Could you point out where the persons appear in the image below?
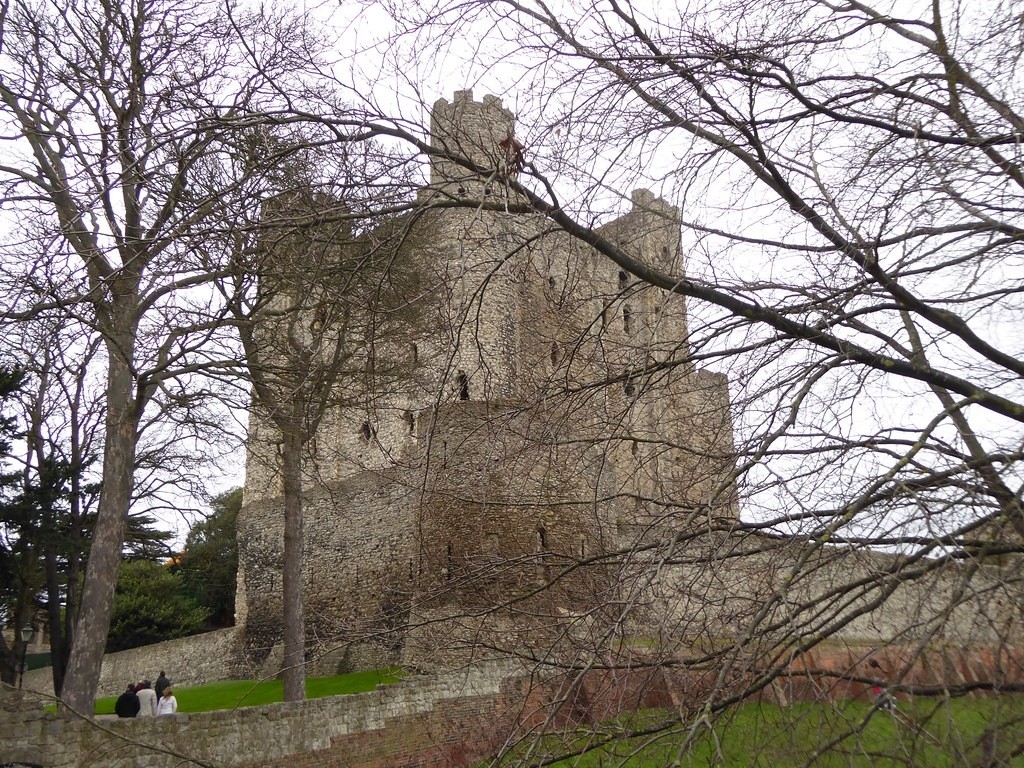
[115,671,177,718]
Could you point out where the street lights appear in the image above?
[18,622,35,691]
